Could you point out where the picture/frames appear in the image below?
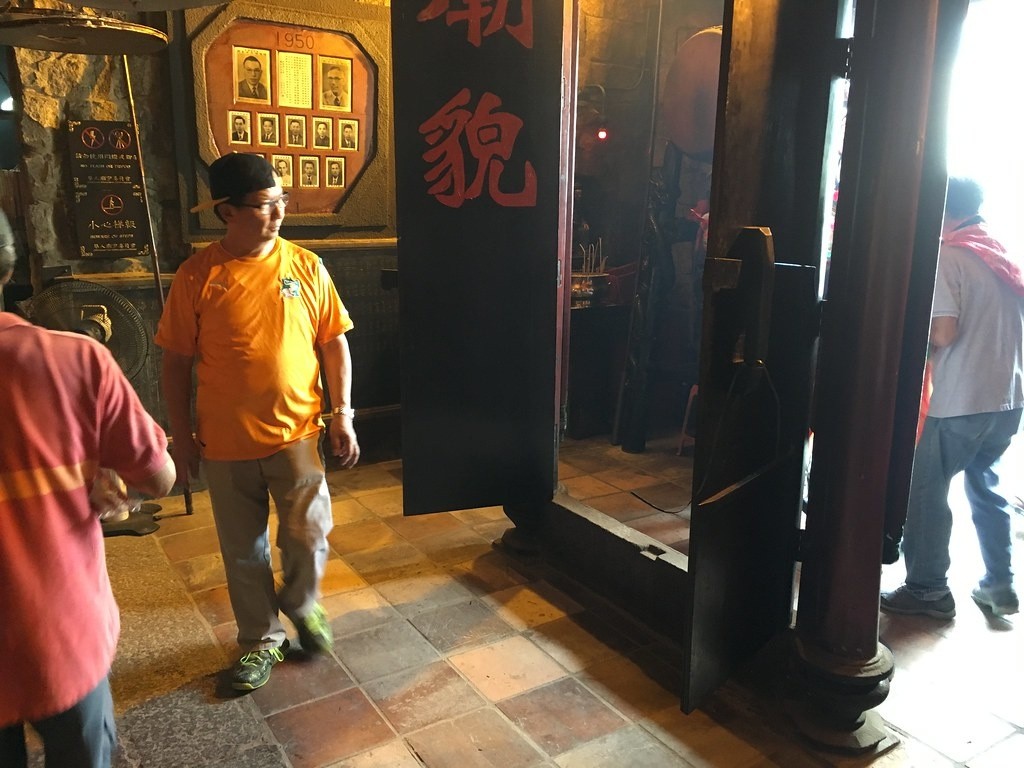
[226,44,359,190]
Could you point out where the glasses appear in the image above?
[235,191,291,215]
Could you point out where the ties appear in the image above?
[307,176,311,183]
[334,177,336,184]
[334,94,339,105]
[347,139,350,147]
[239,132,242,140]
[252,87,257,97]
[266,134,270,139]
[294,135,298,143]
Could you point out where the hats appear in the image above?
[188,151,279,213]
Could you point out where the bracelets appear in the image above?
[330,406,355,419]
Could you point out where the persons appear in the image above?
[276,160,290,183]
[329,162,342,185]
[342,124,355,148]
[153,153,360,690]
[316,122,329,146]
[239,55,266,99]
[0,210,177,768]
[289,120,303,145]
[262,118,276,143]
[322,67,347,107]
[303,161,317,185]
[232,116,248,141]
[879,174,1024,620]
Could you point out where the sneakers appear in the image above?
[969,587,1020,616]
[880,584,956,620]
[231,639,291,692]
[276,591,334,655]
